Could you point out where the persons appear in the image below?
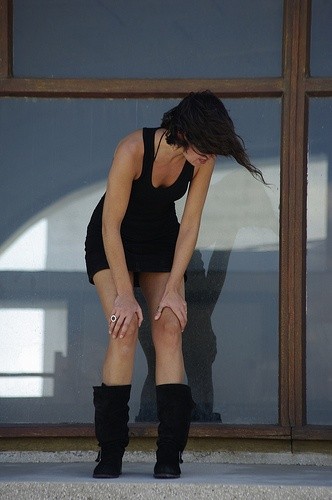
[85,90,266,479]
[134,168,278,423]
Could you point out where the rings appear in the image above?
[111,315,116,322]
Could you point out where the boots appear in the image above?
[153,384,192,478]
[92,383,132,478]
